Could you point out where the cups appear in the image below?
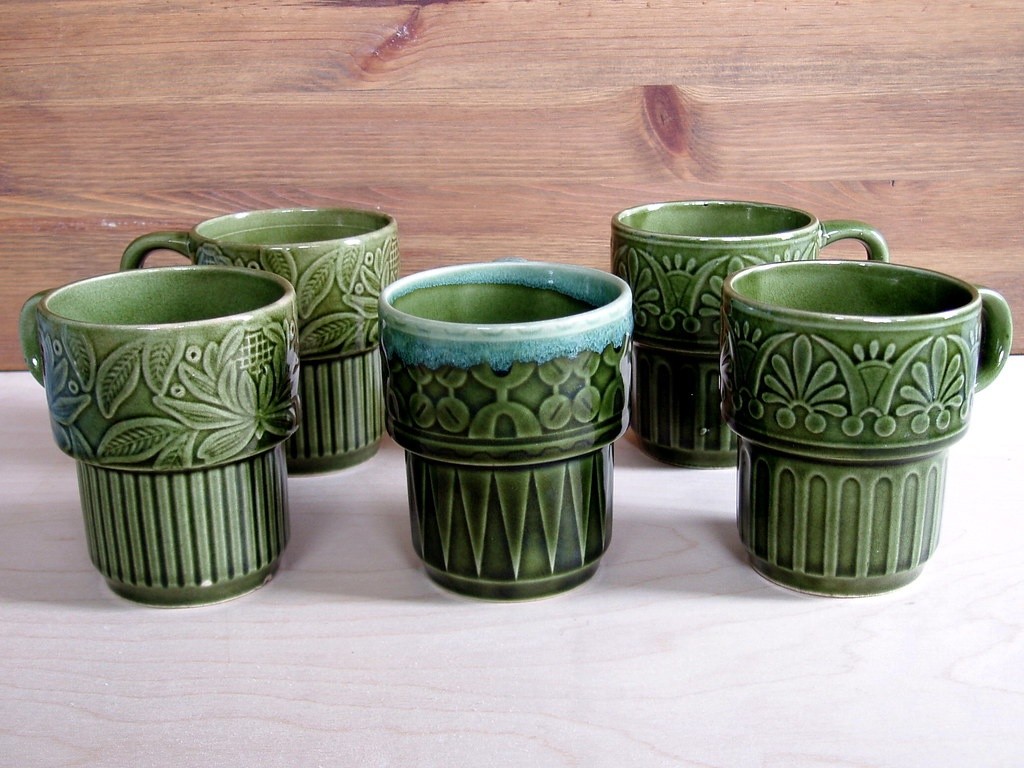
[121,210,400,476]
[607,201,890,469]
[379,259,636,598]
[723,260,1013,596]
[16,268,302,606]
